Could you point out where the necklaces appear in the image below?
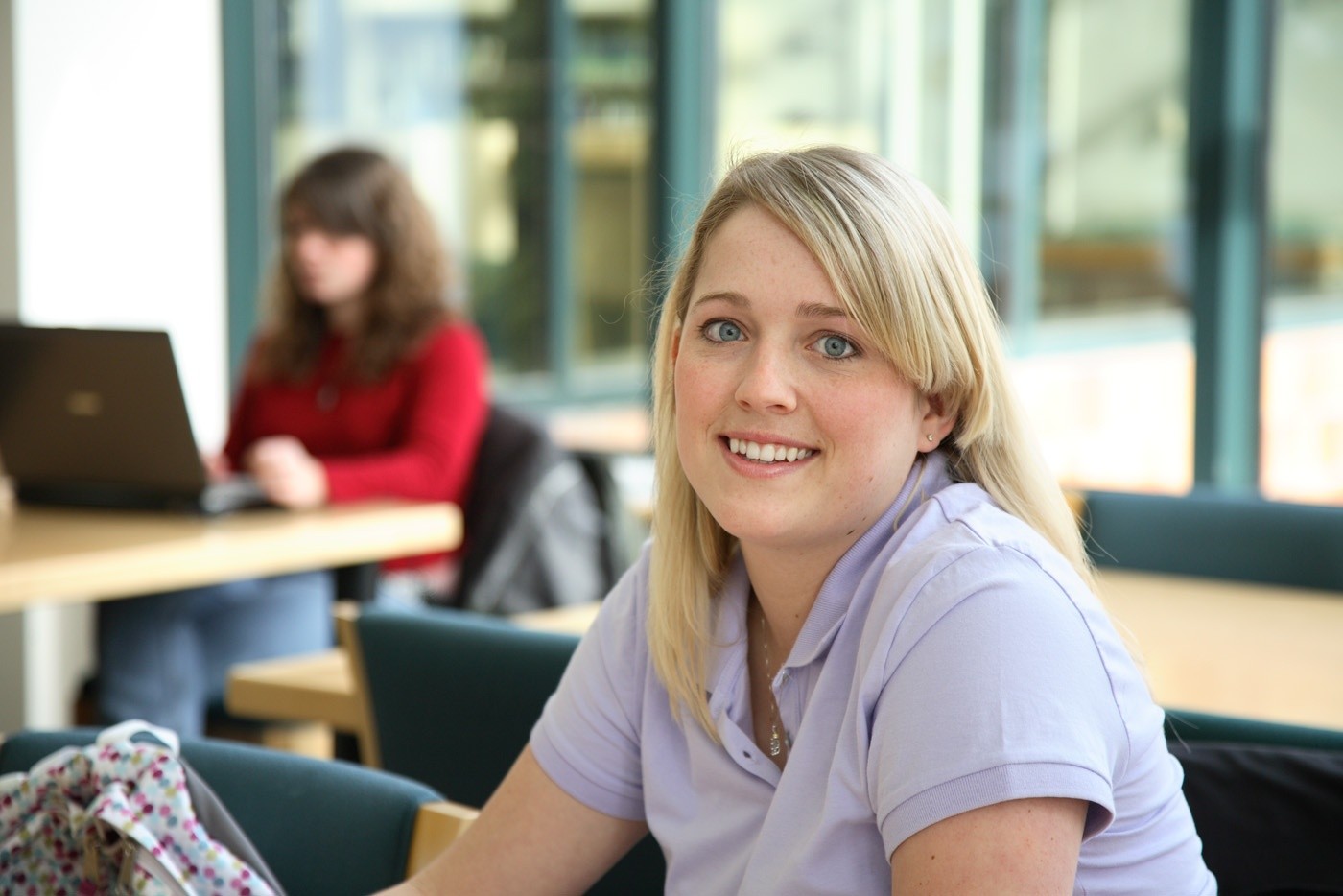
[761,611,781,758]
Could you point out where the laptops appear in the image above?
[0,322,267,518]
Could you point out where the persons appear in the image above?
[95,150,493,737]
[378,145,1218,896]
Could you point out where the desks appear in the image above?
[226,569,1343,778]
[0,476,464,620]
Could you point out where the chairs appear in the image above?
[1158,706,1343,896]
[328,597,578,813]
[354,407,596,608]
[1061,474,1343,596]
[0,727,480,896]
